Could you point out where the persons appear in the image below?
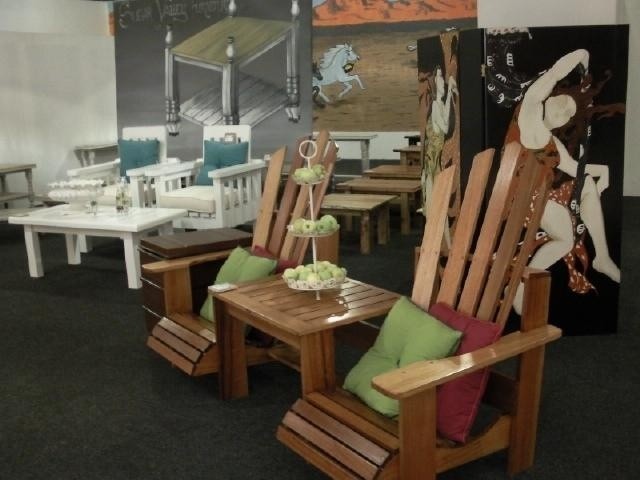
[497,48,620,316]
[420,66,459,249]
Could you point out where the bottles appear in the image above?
[115,177,129,213]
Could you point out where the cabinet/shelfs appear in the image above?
[164,0,301,137]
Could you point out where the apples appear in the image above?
[291,215,337,235]
[283,259,346,284]
[293,163,326,184]
